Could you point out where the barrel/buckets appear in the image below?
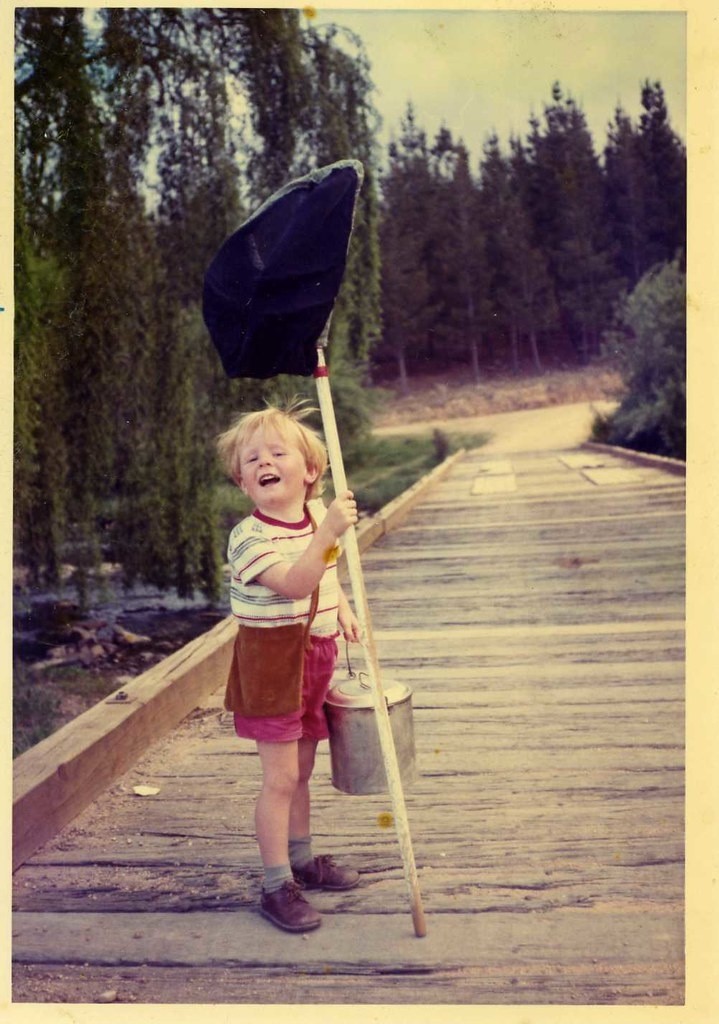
[324,636,415,794]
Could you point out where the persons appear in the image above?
[214,408,361,932]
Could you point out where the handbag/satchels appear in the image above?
[224,622,313,713]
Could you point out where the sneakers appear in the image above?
[259,881,321,931]
[293,853,362,890]
